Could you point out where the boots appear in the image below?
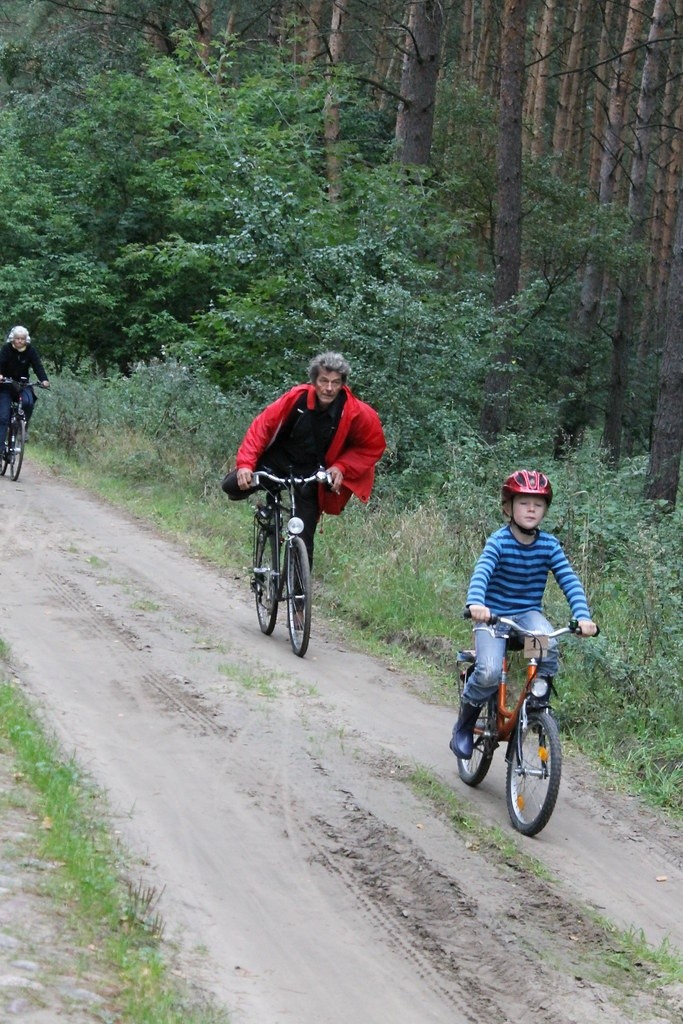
[449,704,481,759]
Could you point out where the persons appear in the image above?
[221,352,386,631]
[449,469,597,759]
[0,326,50,455]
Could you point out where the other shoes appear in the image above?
[290,613,304,628]
[18,431,29,442]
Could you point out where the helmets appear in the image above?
[501,469,553,507]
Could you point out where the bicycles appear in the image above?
[243,471,341,658]
[0,374,51,480]
[454,611,599,837]
